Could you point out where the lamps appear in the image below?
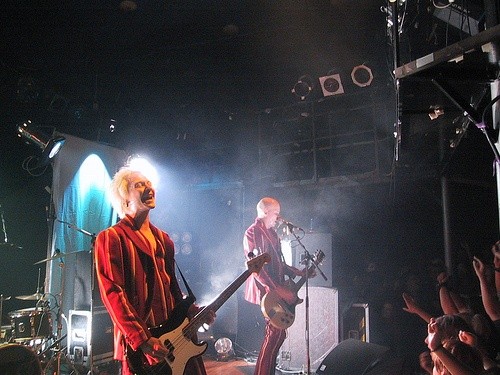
[167,230,194,256]
[214,338,234,362]
[291,75,315,100]
[13,119,66,159]
[319,60,377,96]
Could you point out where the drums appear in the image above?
[8,306,53,340]
[0,343,42,375]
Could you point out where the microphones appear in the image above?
[277,217,300,231]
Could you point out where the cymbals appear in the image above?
[35,248,84,264]
[15,293,46,301]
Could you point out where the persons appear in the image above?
[95,166,217,375]
[243,198,317,375]
[381,240,500,375]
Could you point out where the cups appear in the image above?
[281,351,291,371]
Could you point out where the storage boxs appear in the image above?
[340,303,370,342]
[67,309,114,362]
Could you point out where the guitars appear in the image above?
[125,247,271,375]
[260,249,326,330]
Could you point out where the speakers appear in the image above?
[291,232,348,287]
[74,249,105,311]
[278,287,356,372]
[316,337,404,375]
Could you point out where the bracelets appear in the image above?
[431,345,443,352]
[493,266,500,272]
[436,283,446,289]
